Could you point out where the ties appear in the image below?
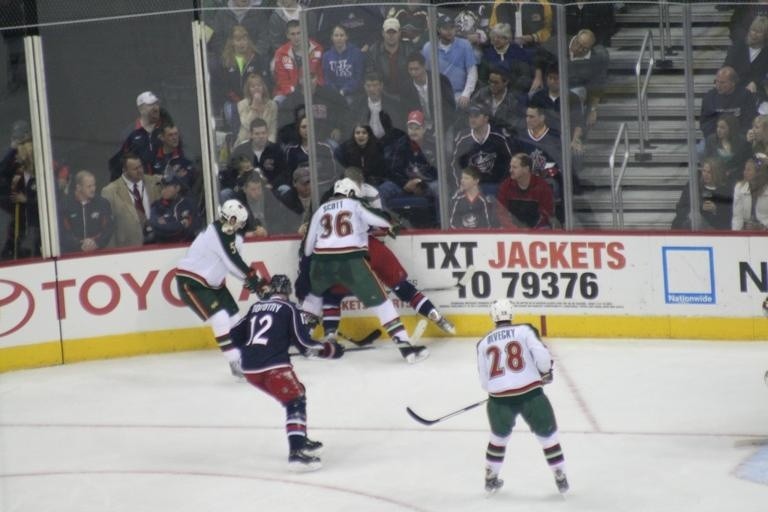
[133,184,148,240]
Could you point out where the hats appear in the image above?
[136,92,159,107]
[465,104,490,119]
[407,111,425,128]
[293,168,311,186]
[382,18,401,33]
[438,15,456,30]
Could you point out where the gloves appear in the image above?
[319,342,346,360]
[540,371,554,385]
[243,270,266,291]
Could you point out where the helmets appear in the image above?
[270,274,292,294]
[220,200,248,230]
[489,299,514,323]
[333,177,361,199]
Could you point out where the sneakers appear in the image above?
[288,449,321,464]
[554,473,569,494]
[435,316,456,335]
[301,438,323,452]
[399,346,426,358]
[484,477,504,491]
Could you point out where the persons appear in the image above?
[230,274,345,471]
[323,228,458,340]
[295,256,330,340]
[670,1,768,234]
[400,3,616,229]
[3,91,206,258]
[211,3,436,239]
[175,201,268,381]
[477,299,569,495]
[299,178,430,363]
